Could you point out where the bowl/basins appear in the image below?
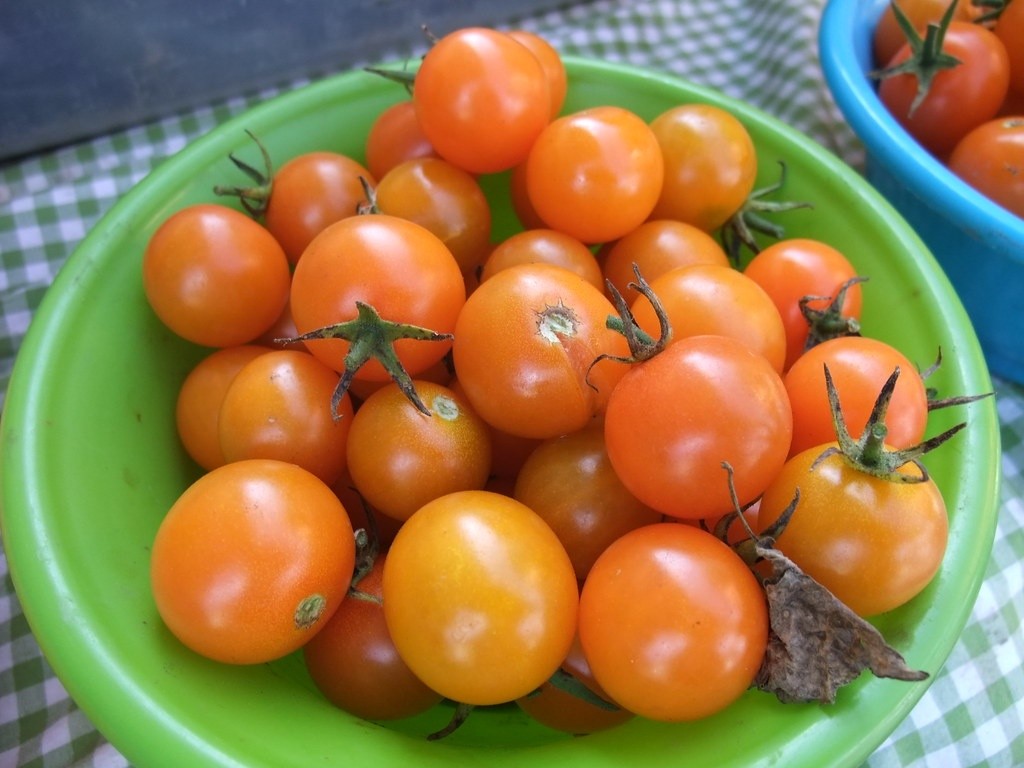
[820,0,1024,390]
[0,55,1001,768]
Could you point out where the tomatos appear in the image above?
[869,0,1024,218]
[144,27,950,736]
